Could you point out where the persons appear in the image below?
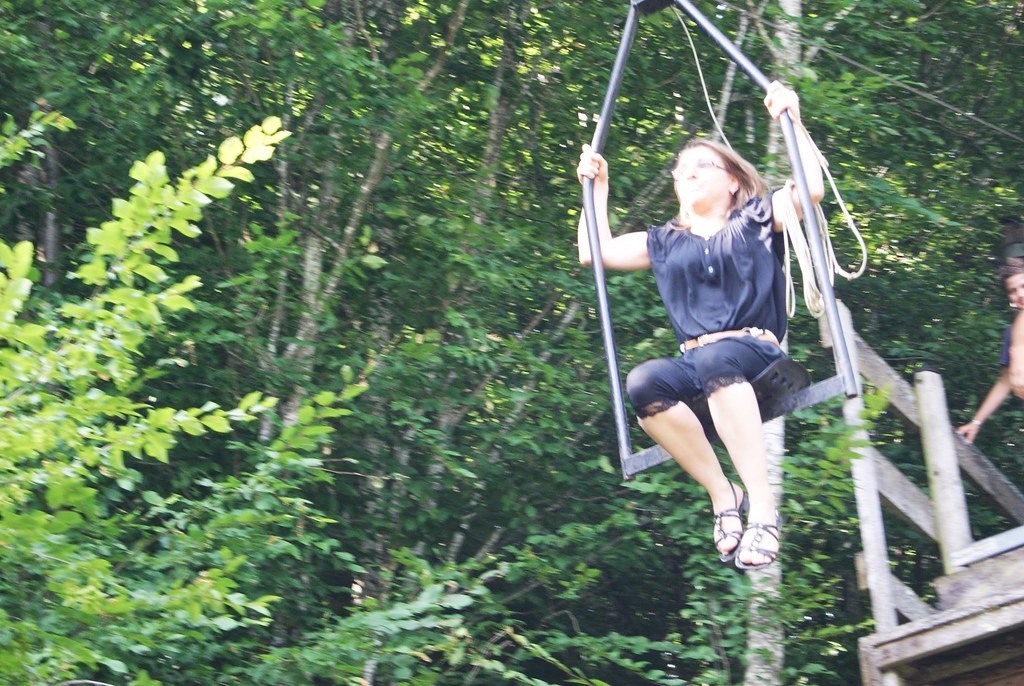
[576,78,826,572]
[950,256,1024,445]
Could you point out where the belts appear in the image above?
[680,326,779,354]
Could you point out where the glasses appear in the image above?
[671,160,733,181]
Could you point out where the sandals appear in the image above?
[714,476,751,563]
[734,503,781,571]
